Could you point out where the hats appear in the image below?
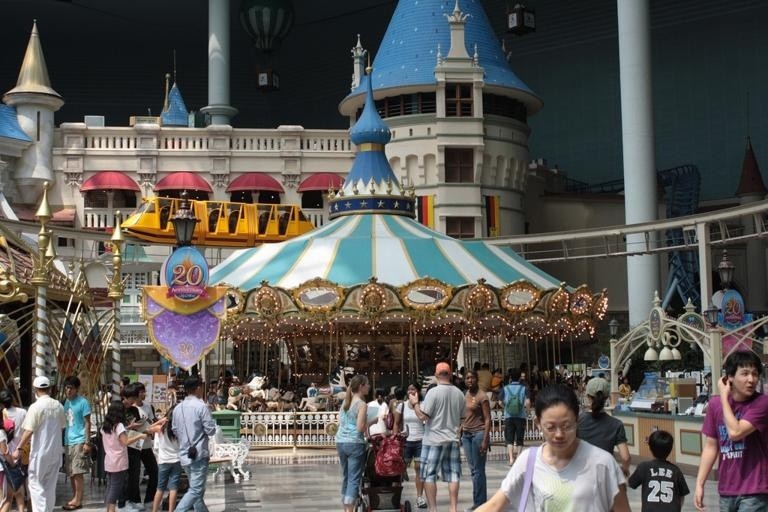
[434,361,454,380]
[585,377,613,398]
[31,375,53,390]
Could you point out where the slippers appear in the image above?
[61,501,84,511]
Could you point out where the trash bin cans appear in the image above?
[208,409,242,470]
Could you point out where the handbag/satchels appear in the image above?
[398,401,406,431]
[1,454,30,493]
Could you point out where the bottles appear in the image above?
[616,399,621,410]
[664,397,678,416]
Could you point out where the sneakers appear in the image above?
[414,496,428,509]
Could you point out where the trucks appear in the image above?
[553,362,586,380]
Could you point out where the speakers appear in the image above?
[84,115,104,126]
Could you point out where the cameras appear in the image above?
[14,459,18,464]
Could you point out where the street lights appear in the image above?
[702,301,722,401]
[167,188,199,252]
[712,247,734,296]
[606,313,621,408]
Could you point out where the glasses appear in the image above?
[540,422,576,432]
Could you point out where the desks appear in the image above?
[207,456,235,465]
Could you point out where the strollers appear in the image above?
[360,422,413,511]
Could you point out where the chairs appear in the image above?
[206,423,253,485]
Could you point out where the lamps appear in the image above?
[643,342,682,363]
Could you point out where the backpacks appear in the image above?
[505,385,527,416]
[374,429,409,478]
